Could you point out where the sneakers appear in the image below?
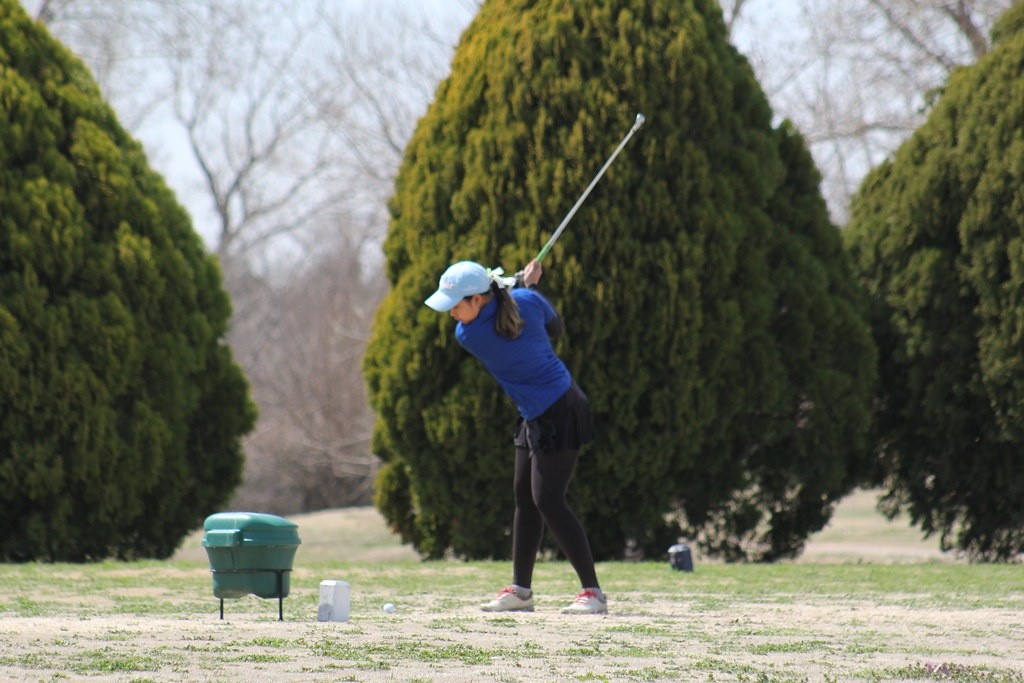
[480,589,534,612]
[561,591,608,614]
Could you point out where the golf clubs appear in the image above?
[514,111,645,286]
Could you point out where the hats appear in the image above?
[424,260,494,313]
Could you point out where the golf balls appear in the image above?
[384,603,395,612]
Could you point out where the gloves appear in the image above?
[513,271,526,288]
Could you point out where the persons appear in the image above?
[423,260,610,617]
